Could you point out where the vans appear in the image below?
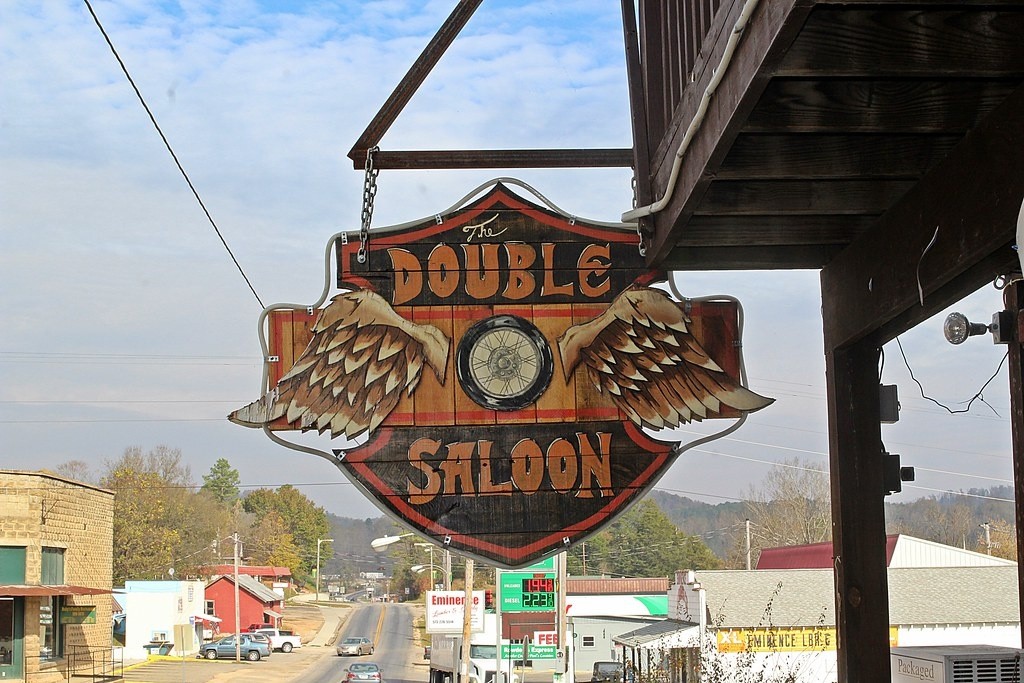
[591,661,623,682]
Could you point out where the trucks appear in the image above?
[430,614,518,683]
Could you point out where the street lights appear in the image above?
[370,533,473,683]
[410,542,452,591]
[316,538,334,605]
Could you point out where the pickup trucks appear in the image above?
[249,629,301,653]
[199,635,270,661]
[242,624,295,636]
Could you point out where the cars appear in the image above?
[337,636,374,656]
[344,664,384,683]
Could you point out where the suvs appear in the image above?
[230,634,272,650]
